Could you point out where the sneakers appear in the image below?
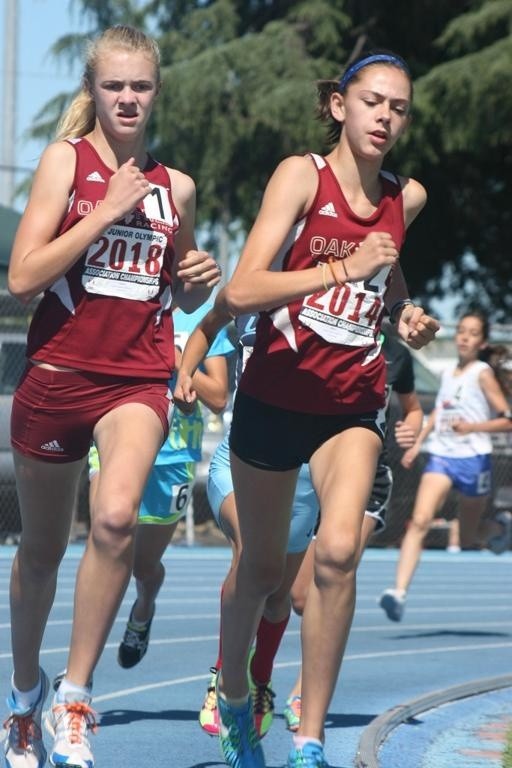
[490,512,512,553]
[199,649,274,738]
[283,692,308,730]
[55,664,67,691]
[5,665,50,767]
[383,586,407,628]
[44,686,95,767]
[116,596,156,669]
[215,669,266,767]
[287,739,330,767]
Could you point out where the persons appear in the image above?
[282,325,426,732]
[2,23,222,766]
[219,42,444,768]
[173,281,321,742]
[85,290,235,668]
[375,309,512,624]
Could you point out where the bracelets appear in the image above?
[389,298,415,328]
[328,255,340,286]
[341,256,349,283]
[321,262,328,290]
